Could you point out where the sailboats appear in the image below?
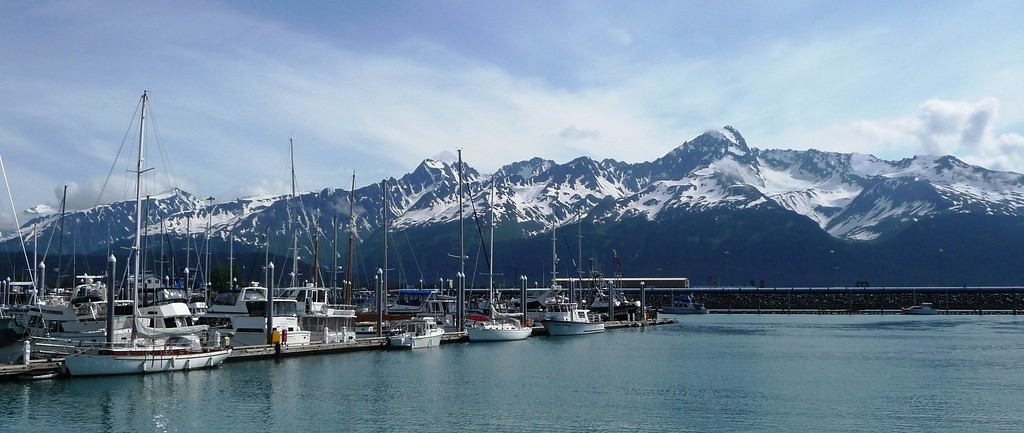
[0,90,675,376]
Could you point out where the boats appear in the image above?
[662,301,708,314]
[901,303,939,315]
